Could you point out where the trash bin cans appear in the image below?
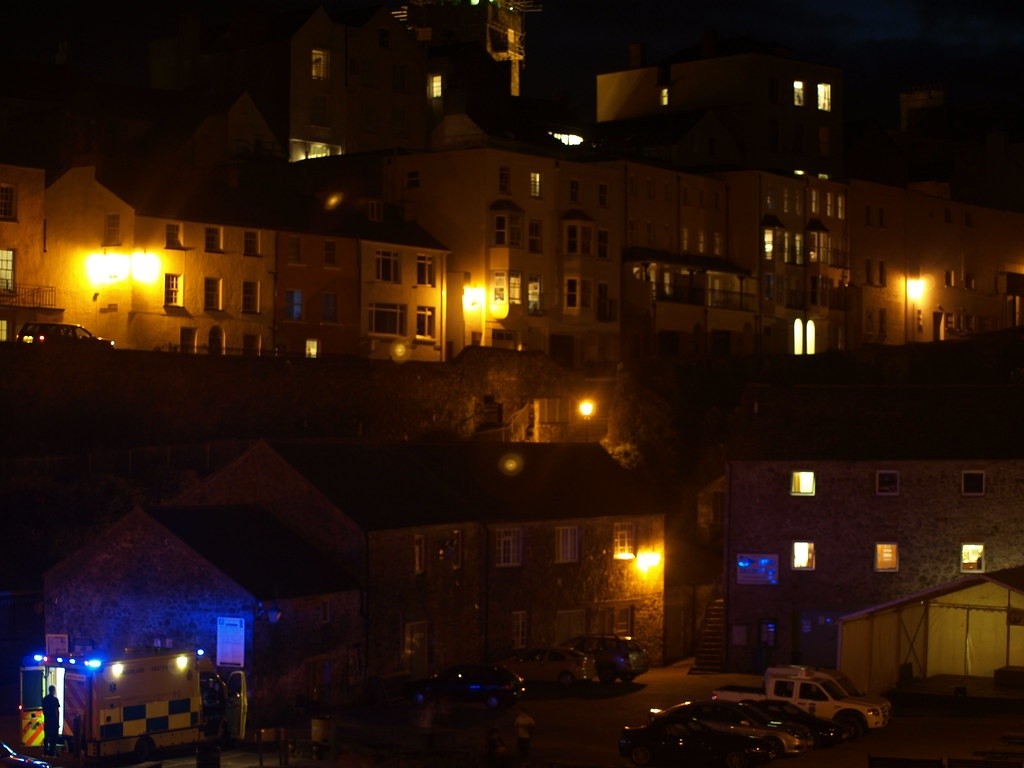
[195,746,222,768]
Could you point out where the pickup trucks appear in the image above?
[710,661,896,746]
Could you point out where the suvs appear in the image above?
[552,632,652,684]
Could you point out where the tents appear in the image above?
[834,566,1024,692]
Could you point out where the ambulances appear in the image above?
[17,636,248,764]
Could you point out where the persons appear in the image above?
[42,685,60,757]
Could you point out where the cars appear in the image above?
[618,708,779,768]
[0,742,52,768]
[650,696,816,760]
[399,665,526,713]
[497,644,594,690]
[739,698,842,750]
